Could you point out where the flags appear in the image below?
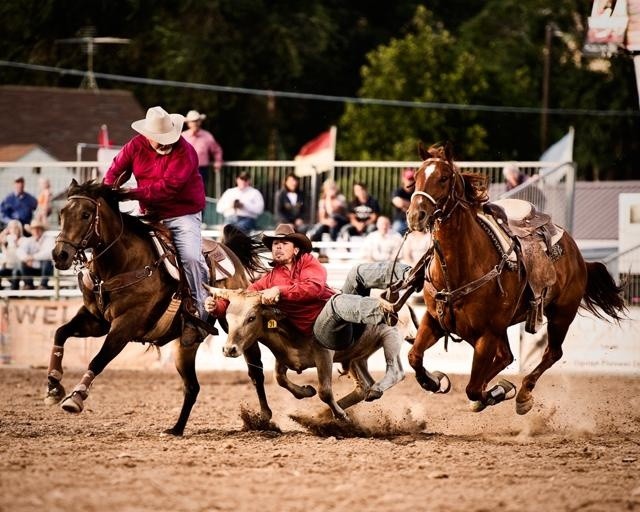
[293,125,336,178]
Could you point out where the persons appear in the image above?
[502,164,546,211]
[216,171,433,266]
[204,223,432,351]
[180,109,224,197]
[0,179,54,290]
[103,106,209,347]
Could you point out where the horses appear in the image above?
[44,178,272,437]
[405,141,636,416]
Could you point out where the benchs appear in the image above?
[1,201,364,289]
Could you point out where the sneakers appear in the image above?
[410,261,425,292]
[181,325,199,346]
[383,286,399,326]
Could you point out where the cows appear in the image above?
[200,282,419,428]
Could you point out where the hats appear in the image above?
[262,224,312,262]
[131,106,184,146]
[184,110,207,122]
